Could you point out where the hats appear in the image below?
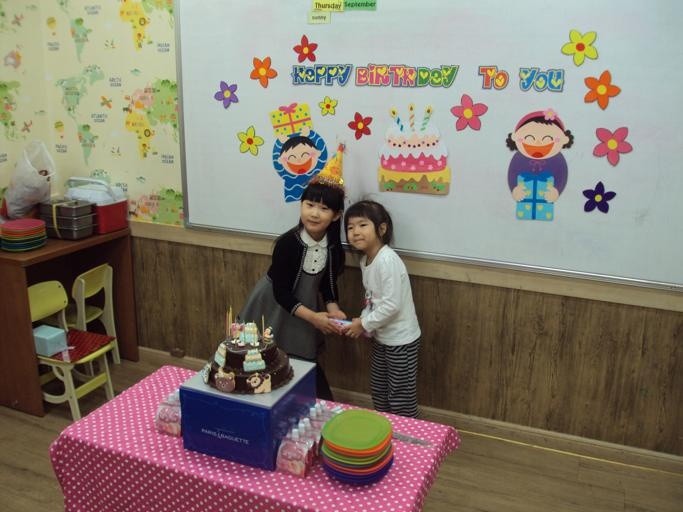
[309,143,345,191]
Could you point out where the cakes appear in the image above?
[377,124,450,196]
[206,335,293,394]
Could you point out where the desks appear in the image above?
[49,365,462,512]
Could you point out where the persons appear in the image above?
[344,200,421,417]
[235,176,346,400]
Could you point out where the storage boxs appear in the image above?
[179,356,315,472]
[65,177,128,235]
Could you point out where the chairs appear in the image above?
[42,263,121,376]
[27,281,117,421]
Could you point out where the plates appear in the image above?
[319,409,395,485]
[0,219,47,252]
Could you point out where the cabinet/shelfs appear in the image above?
[0,228,139,417]
